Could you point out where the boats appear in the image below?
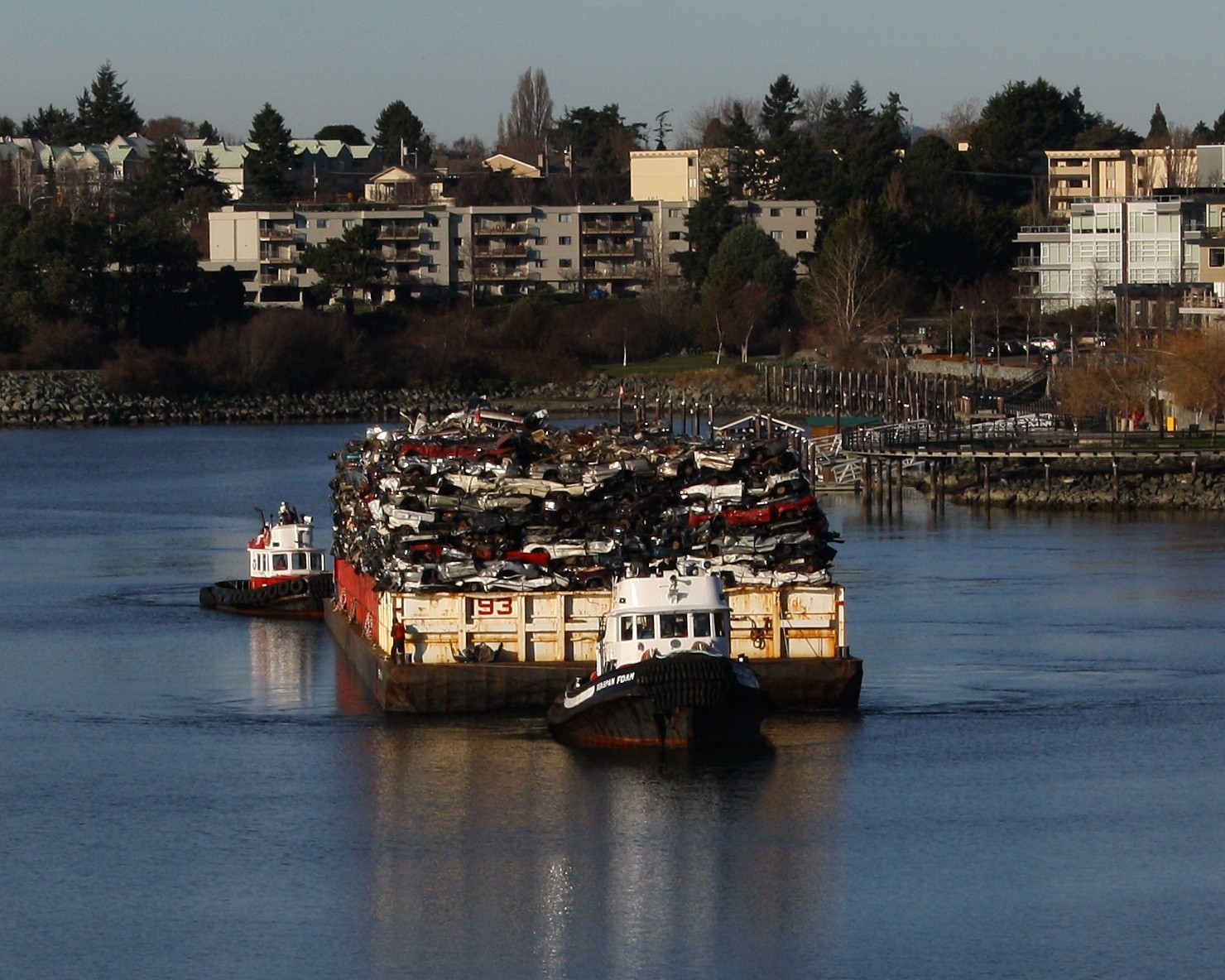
[330,548,862,717]
[563,534,762,749]
[198,499,333,619]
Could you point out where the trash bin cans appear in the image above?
[1121,419,1129,431]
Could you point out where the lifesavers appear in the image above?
[219,588,263,606]
[259,588,267,602]
[278,581,290,596]
[267,584,277,599]
[290,577,307,595]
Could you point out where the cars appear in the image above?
[1029,338,1058,351]
[991,340,1028,353]
[326,401,846,593]
[1078,331,1103,344]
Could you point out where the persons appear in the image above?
[281,506,299,525]
[279,502,286,515]
[1039,346,1050,366]
[801,361,808,376]
